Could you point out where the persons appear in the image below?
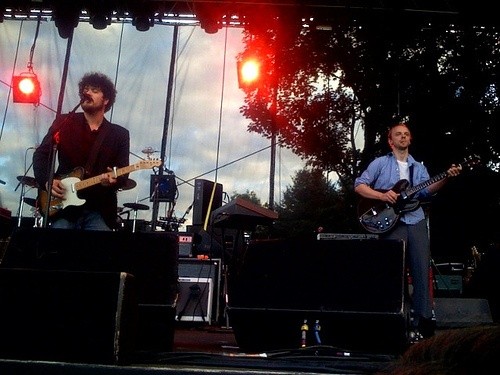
[353,123,463,328]
[385,326,500,375]
[33,72,130,231]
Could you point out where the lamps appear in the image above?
[13,62,41,104]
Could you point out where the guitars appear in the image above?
[358,161,462,234]
[37,158,151,216]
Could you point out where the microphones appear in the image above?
[81,94,91,101]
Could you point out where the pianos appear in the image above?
[211,197,280,229]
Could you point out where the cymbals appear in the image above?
[122,201,150,210]
[22,195,36,209]
[17,173,36,188]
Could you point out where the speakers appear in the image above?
[226,239,407,354]
[0,227,178,363]
[434,298,493,329]
[150,174,176,202]
